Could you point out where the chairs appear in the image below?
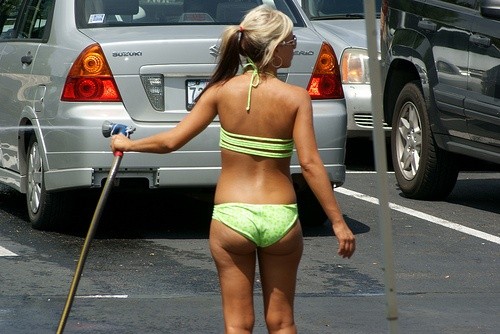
[101,0,139,23]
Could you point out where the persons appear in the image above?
[111,4,357,334]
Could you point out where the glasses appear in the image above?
[277,36,298,49]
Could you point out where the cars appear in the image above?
[297,0,392,151]
[379,0,500,197]
[0,0,347,230]
